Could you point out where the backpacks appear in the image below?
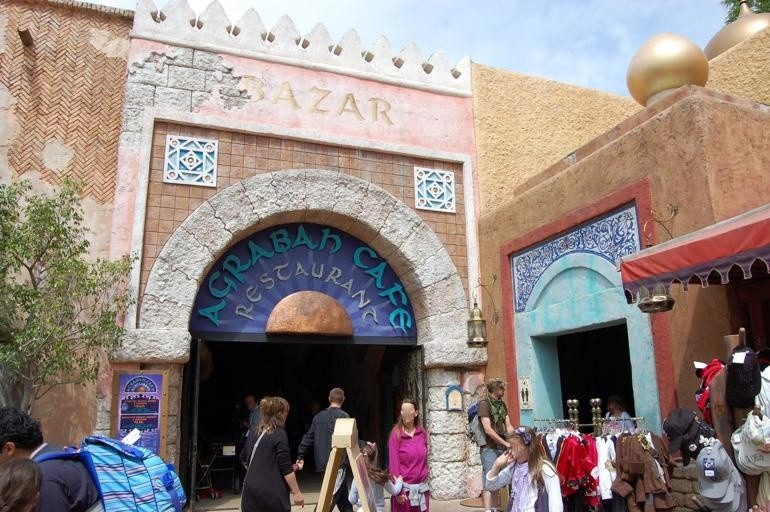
[468,399,486,423]
[33,435,188,512]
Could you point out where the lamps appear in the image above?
[465,272,502,350]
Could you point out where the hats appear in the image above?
[731,410,769,475]
[486,376,505,390]
[697,442,748,511]
[681,416,716,466]
[663,408,695,455]
[726,343,761,410]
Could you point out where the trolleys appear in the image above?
[196,451,221,504]
[201,439,241,495]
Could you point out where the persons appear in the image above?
[604,393,636,433]
[346,440,403,511]
[0,405,104,511]
[296,387,353,511]
[240,395,305,511]
[477,376,515,512]
[485,425,564,512]
[237,393,260,471]
[388,397,430,512]
[0,458,43,511]
